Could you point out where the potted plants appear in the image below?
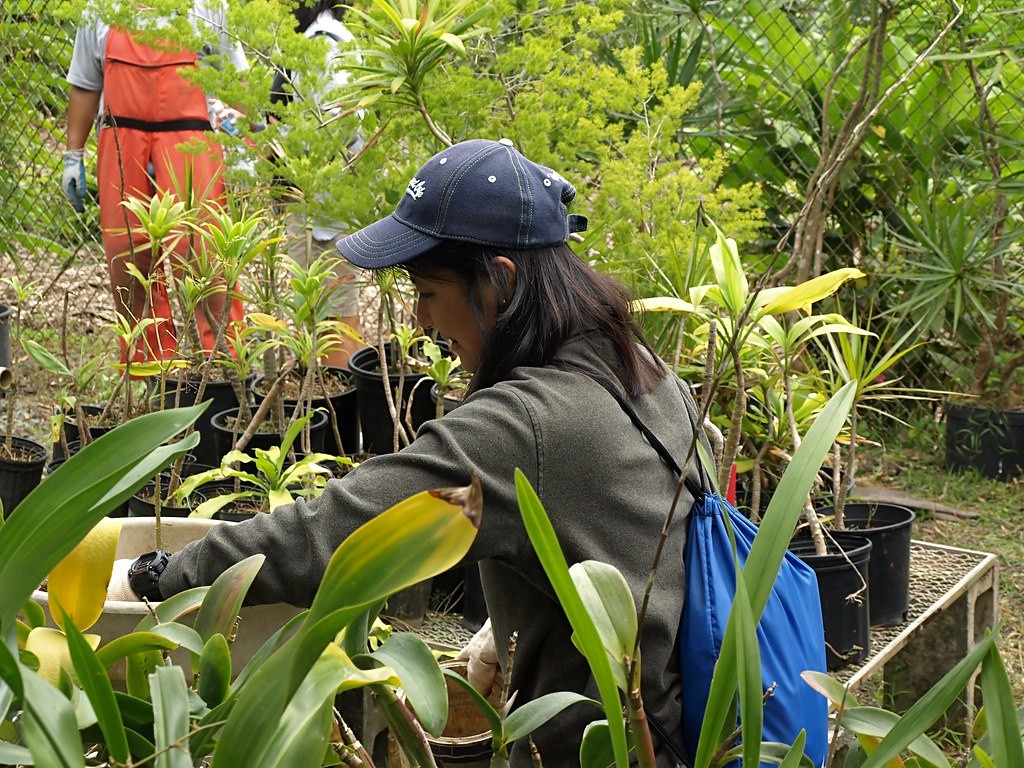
[1,153,474,520]
[17,356,328,701]
[626,213,916,675]
[918,2,1024,480]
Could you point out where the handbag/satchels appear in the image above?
[270,29,344,107]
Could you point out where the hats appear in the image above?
[335,137,589,269]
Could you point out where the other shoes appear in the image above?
[146,375,177,410]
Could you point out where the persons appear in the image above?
[62,0,367,380]
[106,139,826,768]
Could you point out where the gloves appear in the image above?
[62,151,88,214]
[268,164,305,215]
[452,617,508,698]
[104,555,141,602]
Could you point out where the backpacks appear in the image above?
[539,347,830,768]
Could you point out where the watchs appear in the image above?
[126,551,172,607]
